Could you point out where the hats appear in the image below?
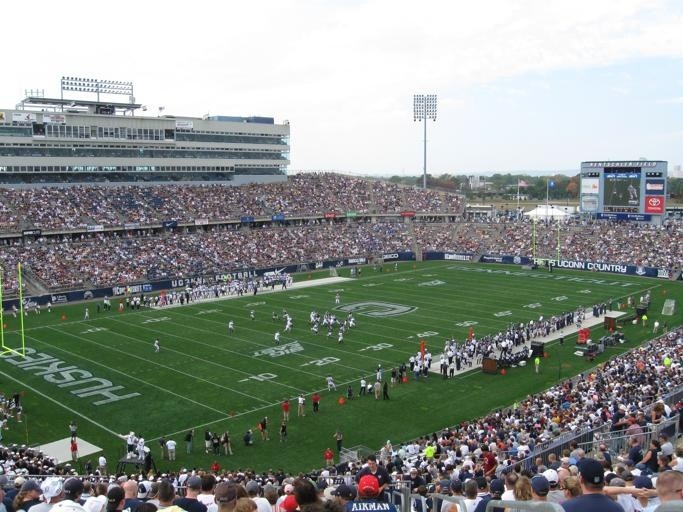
[137,481,152,499]
[22,480,43,495]
[330,484,357,498]
[105,486,125,510]
[214,482,237,503]
[64,477,84,494]
[246,480,259,492]
[357,475,380,496]
[576,458,606,484]
[489,478,505,494]
[539,469,559,486]
[186,477,202,488]
[531,475,549,496]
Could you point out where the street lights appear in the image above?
[411,92,439,189]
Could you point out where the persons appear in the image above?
[345,385,354,399]
[227,320,235,336]
[462,203,683,283]
[325,374,336,393]
[151,338,161,354]
[357,377,366,396]
[272,310,357,346]
[609,185,622,203]
[626,184,637,199]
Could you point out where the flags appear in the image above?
[517,176,528,188]
[546,178,560,193]
